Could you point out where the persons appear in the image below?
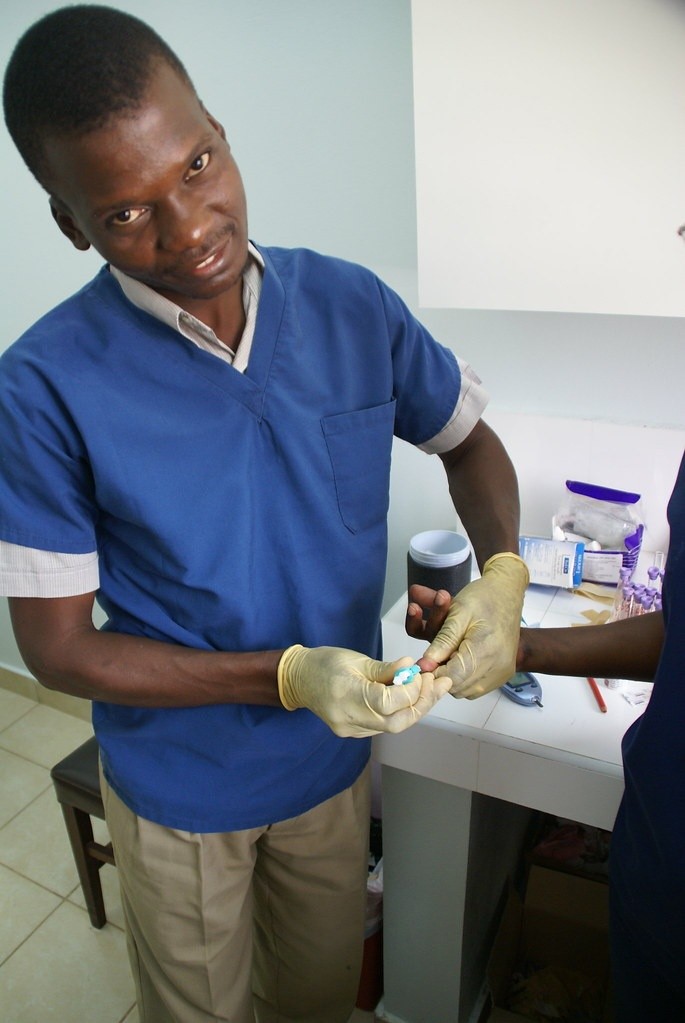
[0,3,528,1023]
[405,448,685,1023]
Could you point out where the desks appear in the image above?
[371,554,685,1023]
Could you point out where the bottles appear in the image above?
[407,530,473,621]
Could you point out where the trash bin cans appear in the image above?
[357,814,384,1012]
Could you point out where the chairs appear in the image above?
[52,730,116,929]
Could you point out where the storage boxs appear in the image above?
[519,535,586,589]
[487,862,607,1023]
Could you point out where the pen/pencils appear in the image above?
[587,676,607,713]
